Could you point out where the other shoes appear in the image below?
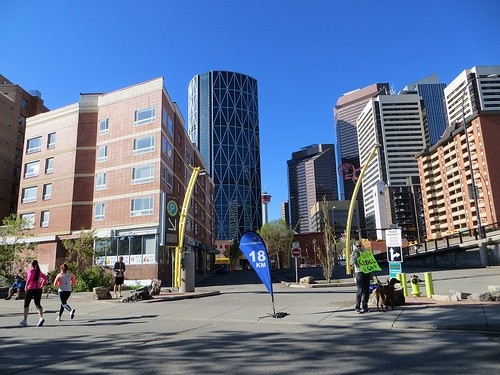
[70,308,75,319]
[56,317,61,321]
[356,310,361,313]
[5,297,10,300]
[16,297,19,300]
[37,318,45,327]
[362,308,371,312]
[20,320,27,325]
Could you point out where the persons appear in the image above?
[54,264,75,321]
[6,274,24,300]
[350,241,371,314]
[20,260,47,327]
[113,256,126,298]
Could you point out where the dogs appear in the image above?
[374,277,401,312]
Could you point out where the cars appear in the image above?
[216,263,231,274]
[234,265,243,271]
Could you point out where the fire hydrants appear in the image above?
[409,275,424,297]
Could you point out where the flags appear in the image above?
[239,231,272,296]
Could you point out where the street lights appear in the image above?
[461,73,498,268]
[345,143,382,278]
[174,164,207,291]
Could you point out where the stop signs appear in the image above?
[291,248,301,257]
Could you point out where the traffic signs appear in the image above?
[385,229,403,262]
